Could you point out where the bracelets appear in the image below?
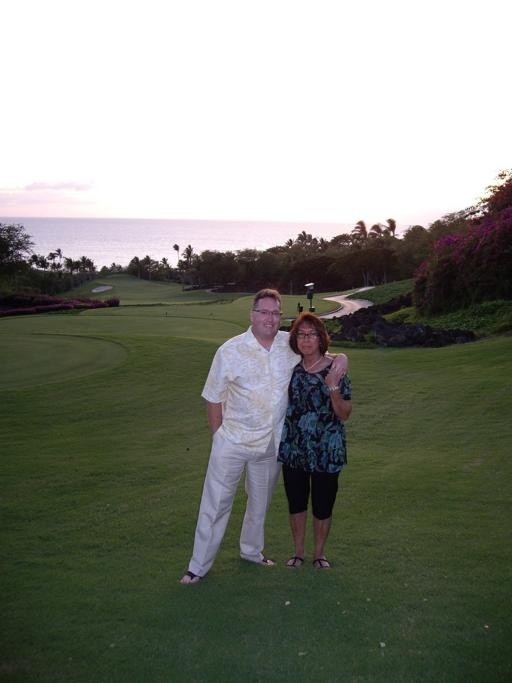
[327,386,340,392]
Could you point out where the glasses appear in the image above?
[293,329,322,340]
[251,308,281,316]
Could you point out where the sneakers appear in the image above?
[312,558,330,568]
[286,556,304,567]
[180,570,201,584]
[239,551,274,566]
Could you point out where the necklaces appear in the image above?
[303,355,324,372]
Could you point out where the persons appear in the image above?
[179,288,349,584]
[277,311,352,570]
